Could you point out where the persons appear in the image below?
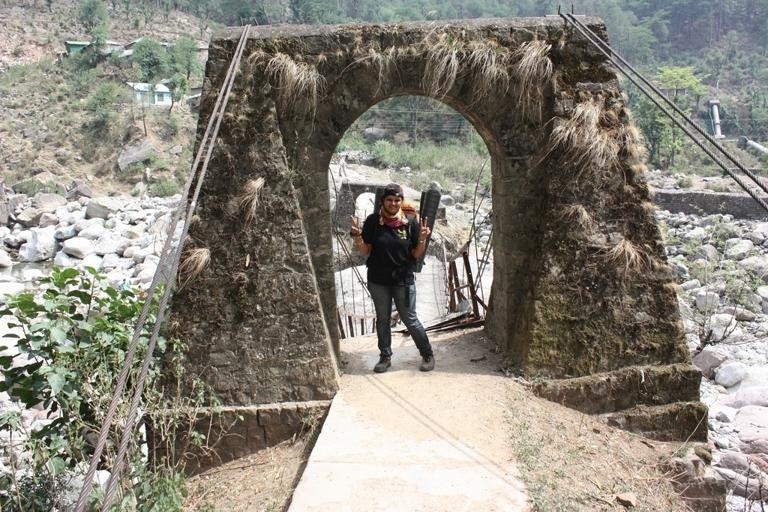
[350,183,436,373]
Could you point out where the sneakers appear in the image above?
[374,354,391,372]
[420,354,434,370]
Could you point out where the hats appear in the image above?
[381,183,404,205]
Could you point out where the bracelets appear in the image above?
[418,239,426,245]
